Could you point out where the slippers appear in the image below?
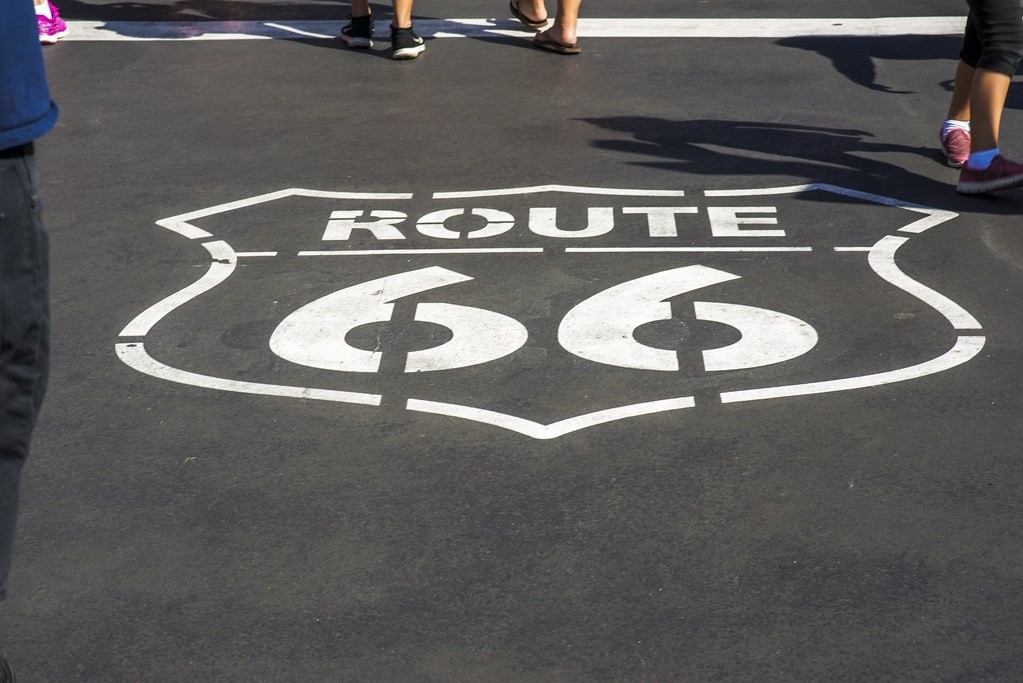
[534,31,581,53]
[510,0,548,27]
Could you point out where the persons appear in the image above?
[510,0,581,54]
[339,0,426,59]
[940,0,1023,196]
[0,1,60,683]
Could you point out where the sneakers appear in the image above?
[389,25,425,59]
[340,14,374,47]
[35,0,68,43]
[940,127,971,167]
[955,156,1023,193]
